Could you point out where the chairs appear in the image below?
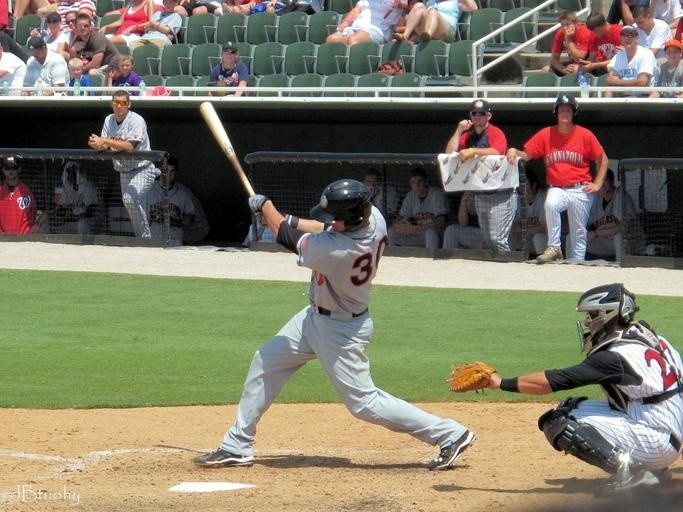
[1,1,635,101]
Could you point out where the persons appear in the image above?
[195,178,476,472]
[447,282,683,498]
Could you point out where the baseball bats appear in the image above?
[200,101,255,198]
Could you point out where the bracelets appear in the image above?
[500,377,520,393]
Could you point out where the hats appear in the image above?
[470,100,489,112]
[664,39,682,50]
[222,42,237,51]
[27,37,43,56]
[3,156,17,167]
[620,25,638,36]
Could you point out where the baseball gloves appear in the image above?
[450,362,496,392]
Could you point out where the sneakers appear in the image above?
[193,448,256,467]
[430,429,477,471]
[608,467,672,493]
[536,247,563,264]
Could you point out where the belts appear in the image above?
[318,306,369,319]
[669,433,681,453]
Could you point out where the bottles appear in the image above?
[35,77,43,96]
[577,71,588,85]
[138,79,146,96]
[72,79,82,96]
[2,79,8,96]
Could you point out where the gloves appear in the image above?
[248,194,272,225]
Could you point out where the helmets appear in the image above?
[309,178,373,227]
[575,283,640,353]
[554,95,580,116]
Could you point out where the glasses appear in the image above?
[112,100,127,106]
[5,166,18,171]
[623,32,634,39]
[471,111,487,117]
